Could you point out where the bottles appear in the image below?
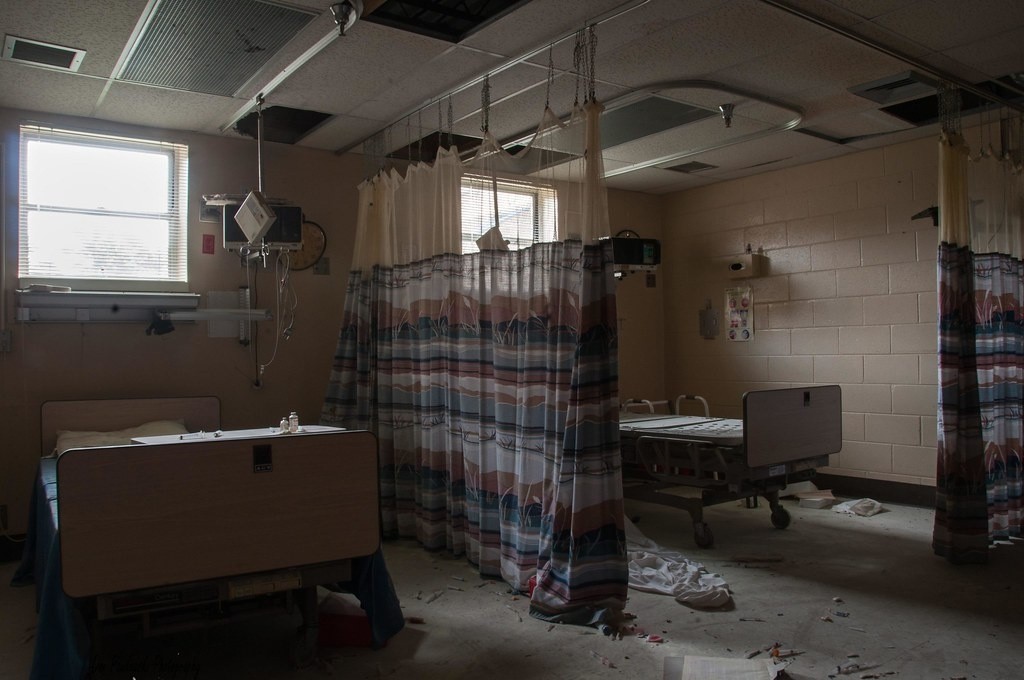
[279,417,289,435]
[289,412,298,433]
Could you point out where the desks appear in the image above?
[131,423,346,444]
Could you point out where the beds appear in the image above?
[9,397,406,680]
[618,385,842,550]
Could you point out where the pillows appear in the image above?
[55,418,190,456]
